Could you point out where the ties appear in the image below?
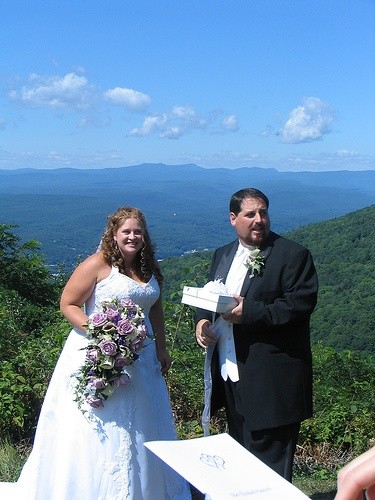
[224,249,249,383]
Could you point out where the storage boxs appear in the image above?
[181,285,245,314]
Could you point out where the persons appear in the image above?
[16,205,191,499]
[192,187,320,483]
[332,446,375,500]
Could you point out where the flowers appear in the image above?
[244,249,265,278]
[70,293,156,416]
